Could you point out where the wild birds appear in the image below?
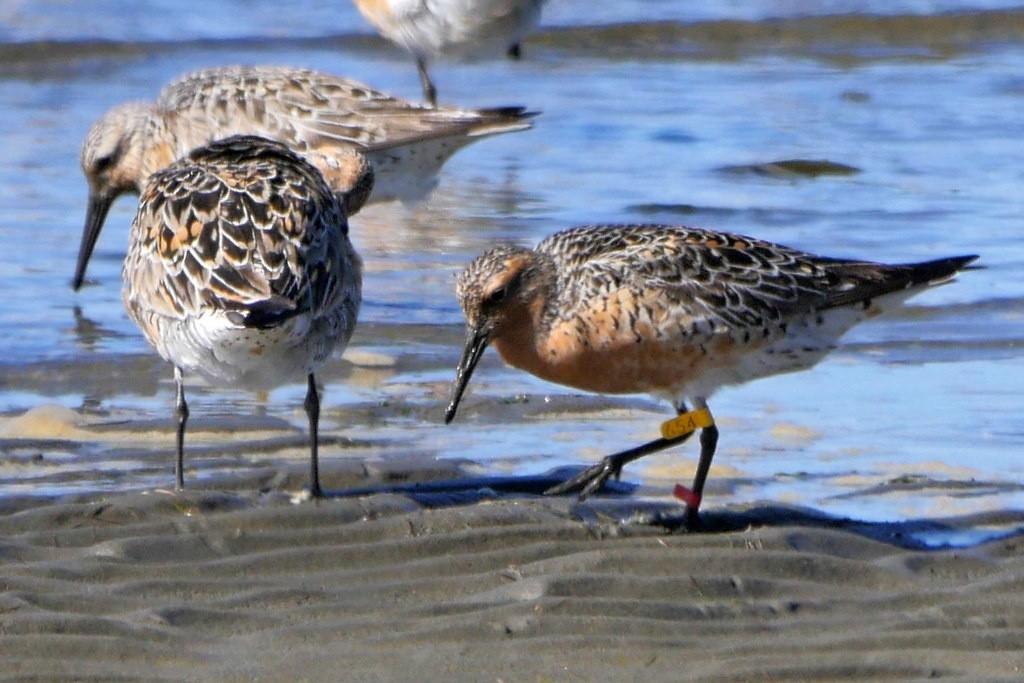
[71,65,544,292]
[121,134,363,501]
[352,0,542,105]
[444,224,980,533]
